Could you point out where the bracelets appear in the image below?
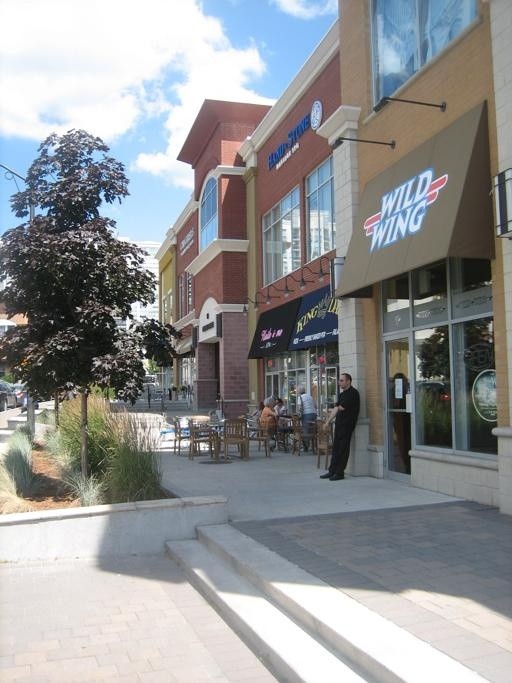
[326,417,333,424]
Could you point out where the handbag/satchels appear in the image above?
[298,395,304,418]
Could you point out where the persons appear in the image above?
[273,397,286,420]
[320,372,359,480]
[296,384,317,451]
[260,397,283,453]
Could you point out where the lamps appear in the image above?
[373,96,446,114]
[490,168,512,240]
[329,136,395,151]
[241,254,329,317]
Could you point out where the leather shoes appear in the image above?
[319,472,344,481]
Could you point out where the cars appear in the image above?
[419,380,451,404]
[0,379,27,412]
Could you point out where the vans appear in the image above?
[138,374,159,394]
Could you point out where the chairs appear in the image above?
[173,410,334,471]
